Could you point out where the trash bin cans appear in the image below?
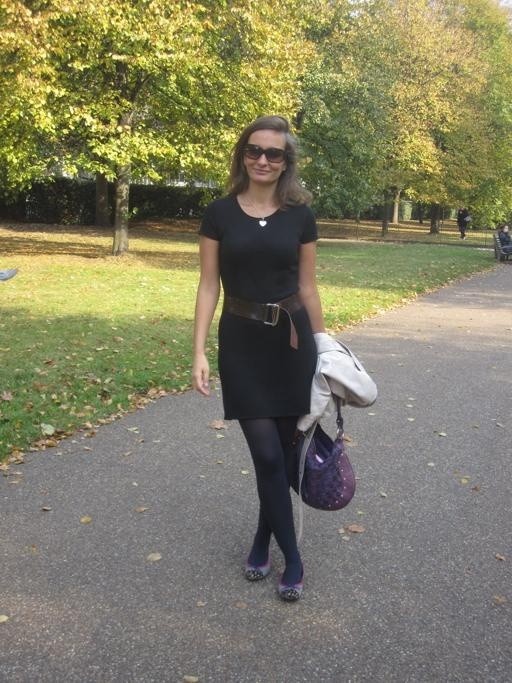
[399,201,413,221]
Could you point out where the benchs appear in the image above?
[493,233,512,262]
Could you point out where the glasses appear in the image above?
[242,142,288,163]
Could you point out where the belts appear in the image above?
[223,287,304,351]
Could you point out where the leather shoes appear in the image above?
[278,561,304,600]
[244,537,270,580]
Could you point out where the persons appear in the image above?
[500,223,512,253]
[457,206,470,241]
[192,115,326,601]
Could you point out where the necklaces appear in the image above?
[243,189,275,227]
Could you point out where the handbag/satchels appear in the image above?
[288,423,356,510]
